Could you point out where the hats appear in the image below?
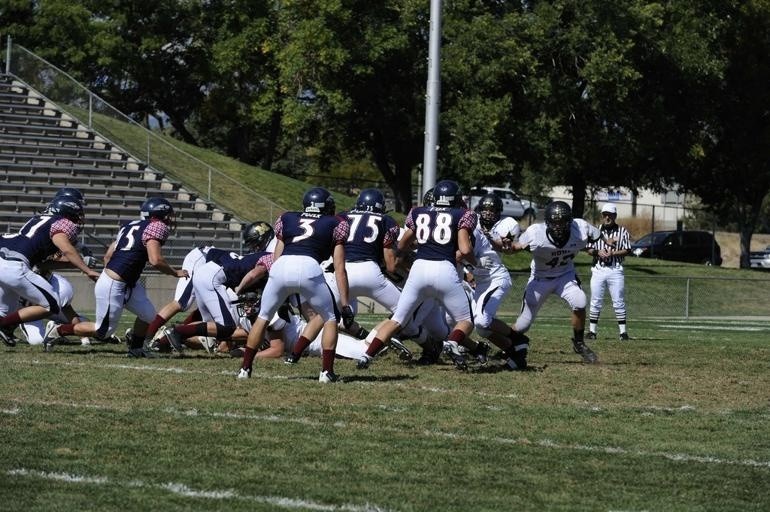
[602,203,616,214]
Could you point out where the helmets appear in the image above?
[233,279,265,317]
[303,180,573,240]
[245,221,274,252]
[44,188,176,235]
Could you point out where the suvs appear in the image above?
[462,187,538,223]
[625,229,723,267]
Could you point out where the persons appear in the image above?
[501,201,620,366]
[579,203,634,343]
[1,180,532,385]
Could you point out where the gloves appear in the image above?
[474,256,494,269]
[341,306,353,329]
[278,302,294,323]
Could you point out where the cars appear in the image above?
[748,244,770,270]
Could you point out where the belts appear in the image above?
[604,262,612,266]
[212,258,230,282]
